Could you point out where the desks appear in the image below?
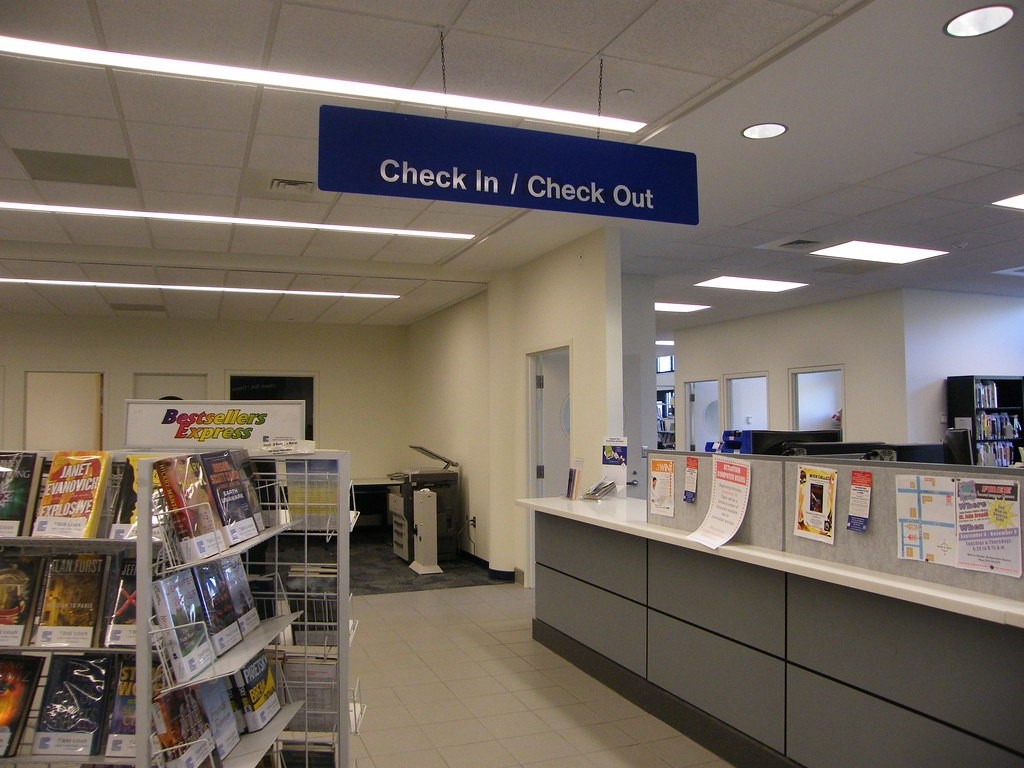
[349,477,405,546]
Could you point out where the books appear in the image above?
[975,379,998,408]
[0,448,286,768]
[245,562,338,641]
[657,390,676,450]
[265,647,337,731]
[974,412,1022,467]
[565,468,579,500]
[250,451,345,530]
[582,475,617,500]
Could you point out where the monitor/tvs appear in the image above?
[752,430,839,456]
[946,428,974,465]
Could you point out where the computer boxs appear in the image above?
[869,444,944,463]
[788,442,885,464]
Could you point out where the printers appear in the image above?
[388,444,459,563]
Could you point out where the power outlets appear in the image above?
[473,516,476,528]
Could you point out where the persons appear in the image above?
[651,477,659,503]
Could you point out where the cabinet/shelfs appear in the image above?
[0,443,366,768]
[658,387,675,450]
[948,374,1024,467]
